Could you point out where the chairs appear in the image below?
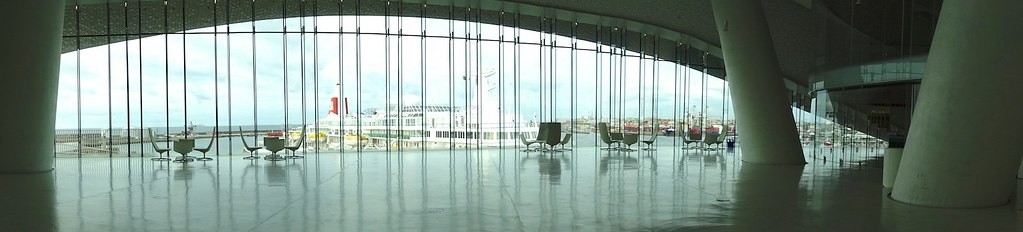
[174,140,192,163]
[558,134,573,151]
[266,137,285,161]
[711,126,728,152]
[639,124,660,150]
[520,134,536,153]
[285,125,306,159]
[598,123,619,151]
[239,126,262,159]
[705,128,717,153]
[610,133,624,150]
[193,126,216,160]
[147,128,172,162]
[622,133,638,153]
[679,123,694,151]
[547,123,565,153]
[263,137,280,159]
[179,138,195,164]
[690,129,702,150]
